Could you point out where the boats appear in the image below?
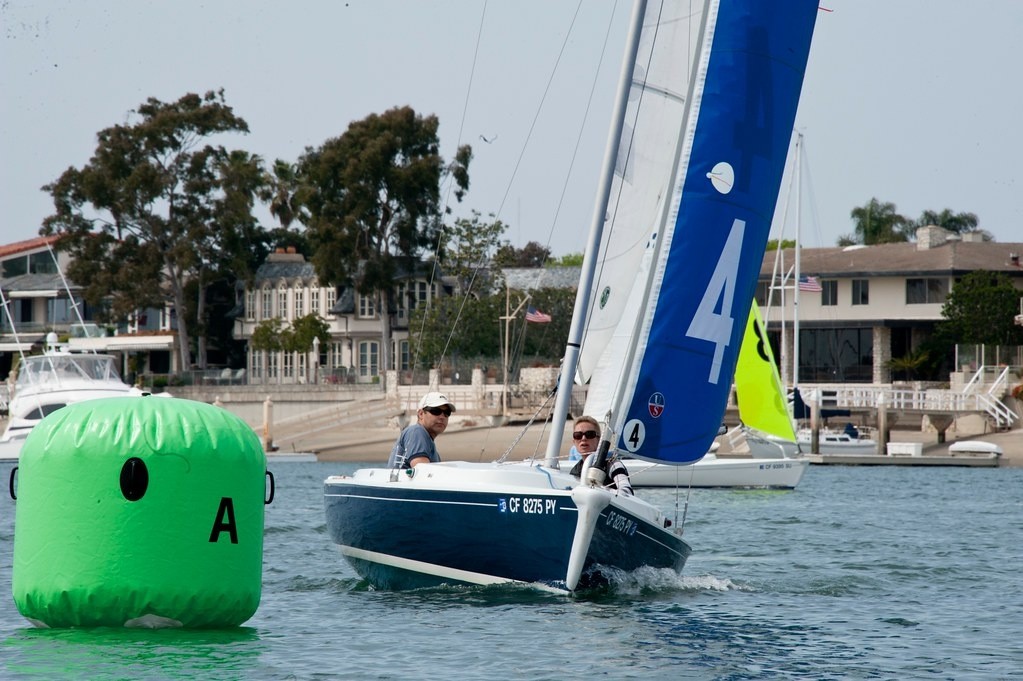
[795,425,1010,467]
[0,239,174,461]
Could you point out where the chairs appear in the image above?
[204,367,246,386]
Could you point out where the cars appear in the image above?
[320,367,356,385]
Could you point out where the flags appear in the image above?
[799,273,823,292]
[525,305,552,323]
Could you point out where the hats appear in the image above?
[417,392,456,412]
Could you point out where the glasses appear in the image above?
[572,430,600,440]
[422,407,452,417]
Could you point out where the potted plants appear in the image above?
[486,367,497,384]
[442,364,452,384]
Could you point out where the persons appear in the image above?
[844,422,858,438]
[387,392,456,469]
[569,416,635,496]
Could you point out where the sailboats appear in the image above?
[323,0,821,598]
[569,128,810,489]
